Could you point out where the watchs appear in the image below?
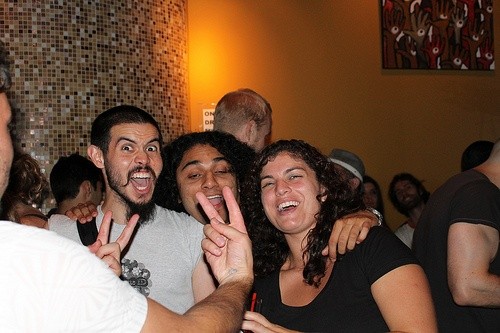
[366,206,383,227]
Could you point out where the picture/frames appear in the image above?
[378,0,496,77]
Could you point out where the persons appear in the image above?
[461,139,495,172]
[355,174,383,216]
[43,151,105,227]
[240,136,442,333]
[0,151,48,230]
[212,86,274,157]
[409,139,500,332]
[62,129,386,262]
[325,147,365,196]
[0,66,256,331]
[42,101,217,317]
[386,170,433,248]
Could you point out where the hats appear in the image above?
[327,148,365,181]
